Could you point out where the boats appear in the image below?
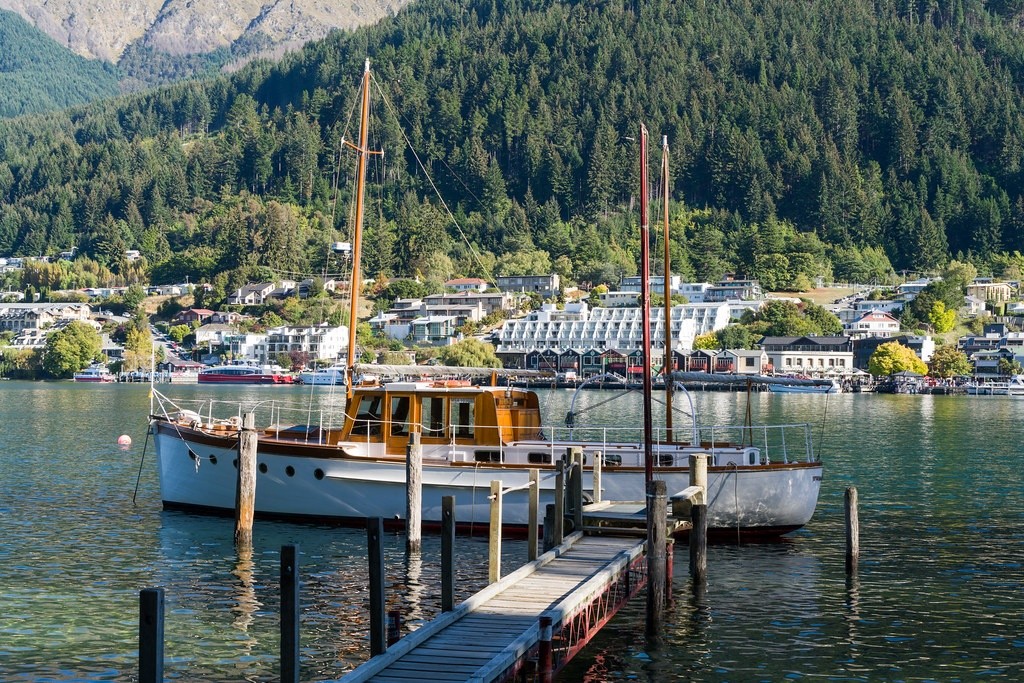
[198,365,295,384]
[966,379,1024,396]
[850,384,882,393]
[73,367,118,383]
[766,383,842,394]
[299,369,346,386]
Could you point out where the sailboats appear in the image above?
[146,56,827,540]
